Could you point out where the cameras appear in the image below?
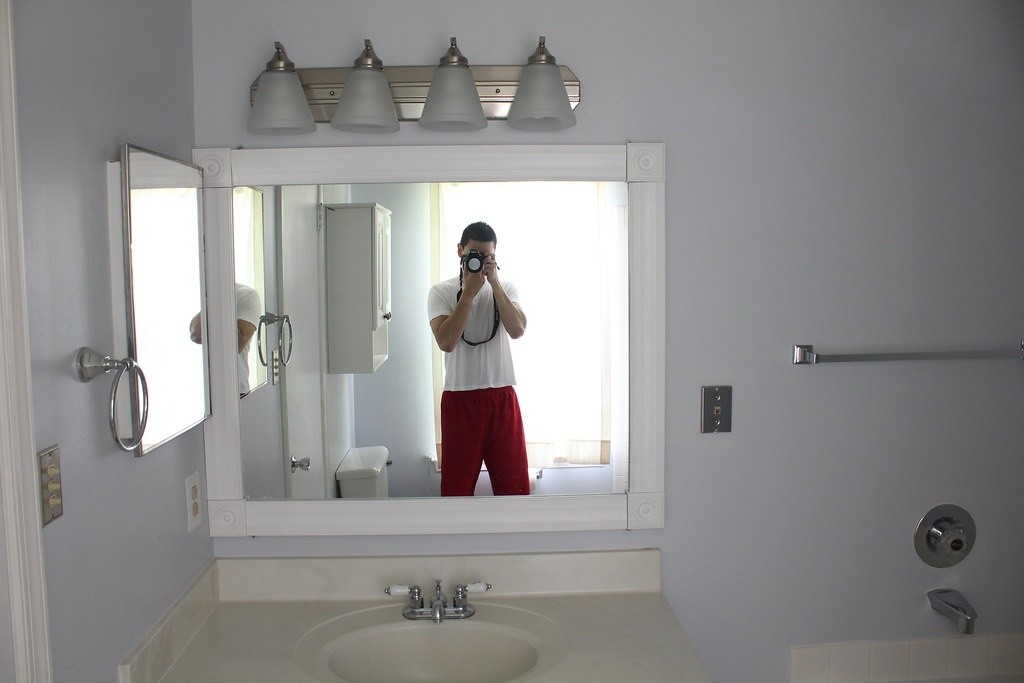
[464,249,489,273]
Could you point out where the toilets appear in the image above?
[333,442,396,502]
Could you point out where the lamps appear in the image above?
[330,39,400,134]
[506,35,577,132]
[417,37,488,131]
[245,41,317,136]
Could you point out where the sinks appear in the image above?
[291,601,572,683]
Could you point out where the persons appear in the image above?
[427,222,530,497]
[190,283,260,396]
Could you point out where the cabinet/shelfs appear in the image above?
[326,202,392,374]
[231,182,631,505]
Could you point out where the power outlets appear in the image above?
[185,469,202,532]
[700,385,733,433]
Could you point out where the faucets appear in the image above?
[926,590,978,638]
[384,577,493,625]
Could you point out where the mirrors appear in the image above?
[120,143,212,456]
[231,186,269,400]
